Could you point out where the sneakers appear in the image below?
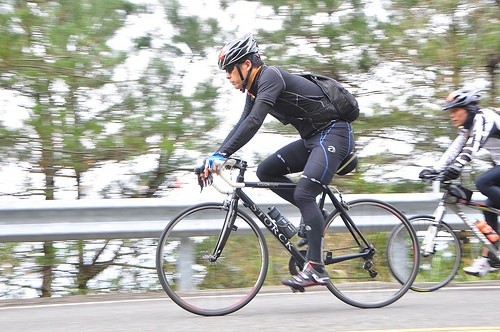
[297,208,328,247]
[282,264,330,286]
[463,255,498,273]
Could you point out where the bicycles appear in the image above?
[385,170,500,293]
[156,152,421,316]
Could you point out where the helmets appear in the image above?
[443,89,481,111]
[218,37,258,69]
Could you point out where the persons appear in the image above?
[420,90,500,276]
[199,33,355,287]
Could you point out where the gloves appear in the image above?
[445,166,461,179]
[419,168,438,179]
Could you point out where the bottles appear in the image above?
[475,218,500,243]
[267,206,297,239]
[298,215,306,238]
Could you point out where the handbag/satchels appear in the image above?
[303,73,359,122]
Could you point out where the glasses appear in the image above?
[225,62,236,73]
